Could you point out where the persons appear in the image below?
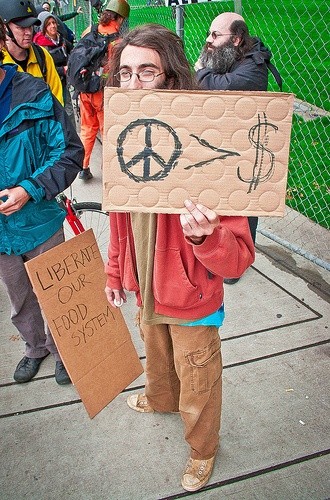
[0,50,85,387]
[66,0,155,180]
[194,13,272,285]
[0,0,77,129]
[104,26,255,492]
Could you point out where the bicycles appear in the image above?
[56,191,112,269]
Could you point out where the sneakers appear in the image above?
[126,392,181,414]
[180,446,219,492]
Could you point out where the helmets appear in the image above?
[0,0,41,28]
[105,0,130,19]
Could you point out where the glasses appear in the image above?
[113,68,165,82]
[206,31,236,39]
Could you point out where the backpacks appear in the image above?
[65,23,123,94]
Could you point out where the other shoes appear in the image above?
[13,350,50,383]
[78,167,93,181]
[223,278,240,285]
[54,360,71,385]
